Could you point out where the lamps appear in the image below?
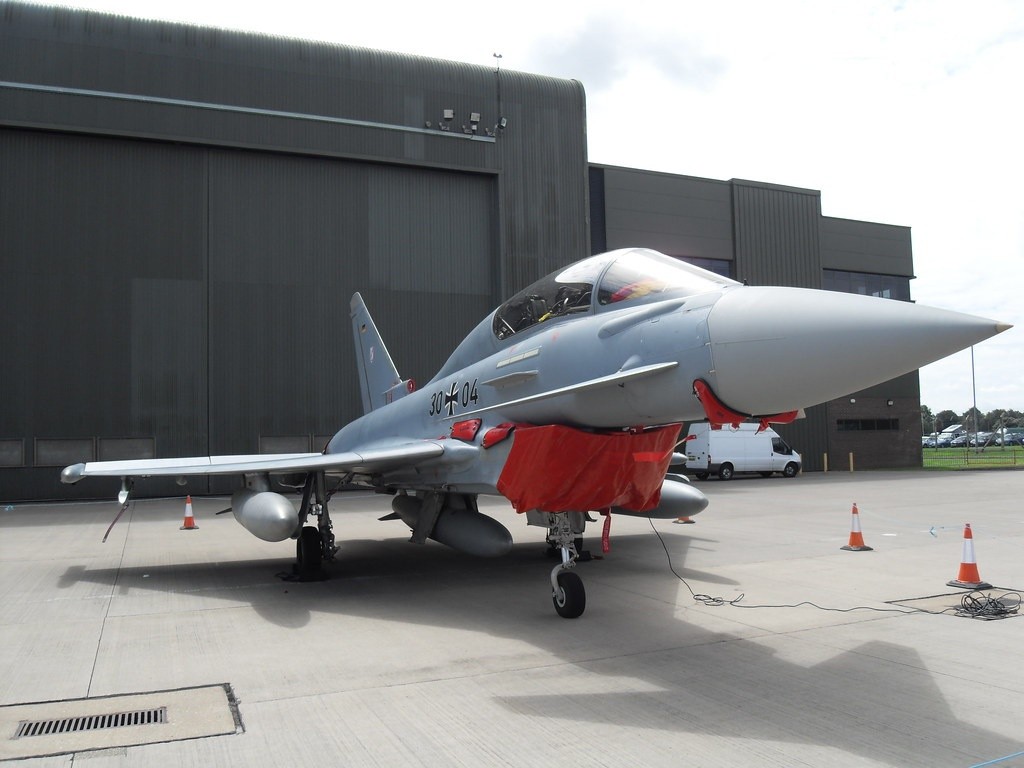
[439,110,453,132]
[486,117,507,136]
[462,112,480,135]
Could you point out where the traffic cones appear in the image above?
[841,503,874,551]
[671,516,696,524]
[945,523,993,590]
[178,494,199,531]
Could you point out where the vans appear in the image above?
[997,427,1024,435]
[941,424,966,440]
[686,422,802,481]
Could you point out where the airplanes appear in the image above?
[59,248,1013,620]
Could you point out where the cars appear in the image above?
[969,432,998,447]
[929,436,953,448]
[995,433,1024,447]
[950,436,974,447]
[921,436,936,448]
